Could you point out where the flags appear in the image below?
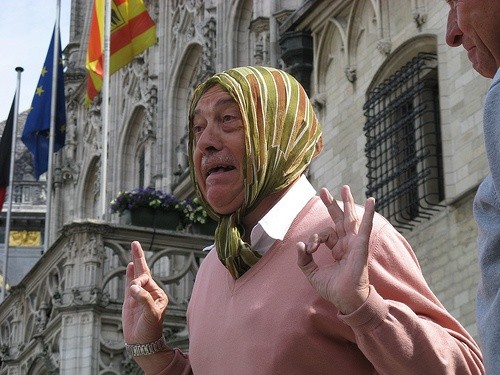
[0,87,16,221]
[22,21,67,183]
[85,0,158,110]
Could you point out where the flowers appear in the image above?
[176,197,206,224]
[111,188,179,217]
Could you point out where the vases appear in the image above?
[129,208,181,231]
[192,223,218,235]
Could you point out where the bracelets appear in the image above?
[123,334,167,359]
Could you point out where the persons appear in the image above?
[445,0,500,375]
[122,66,485,375]
